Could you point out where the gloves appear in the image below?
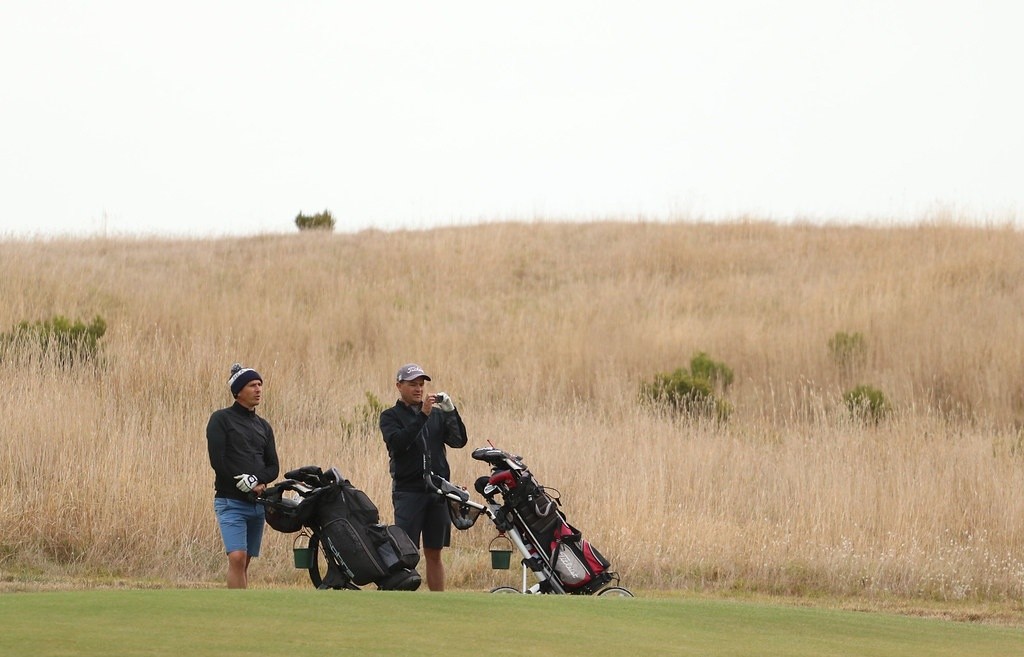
[436,392,455,412]
[234,474,258,492]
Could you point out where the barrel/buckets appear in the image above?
[293,534,314,568]
[489,536,513,569]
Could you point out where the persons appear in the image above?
[379,364,467,591]
[206,363,280,588]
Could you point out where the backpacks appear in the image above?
[295,479,422,591]
[492,473,620,596]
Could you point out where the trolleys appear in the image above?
[425,469,634,598]
[248,485,422,591]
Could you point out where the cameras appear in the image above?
[433,395,443,403]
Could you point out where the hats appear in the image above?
[396,363,431,382]
[228,363,263,399]
